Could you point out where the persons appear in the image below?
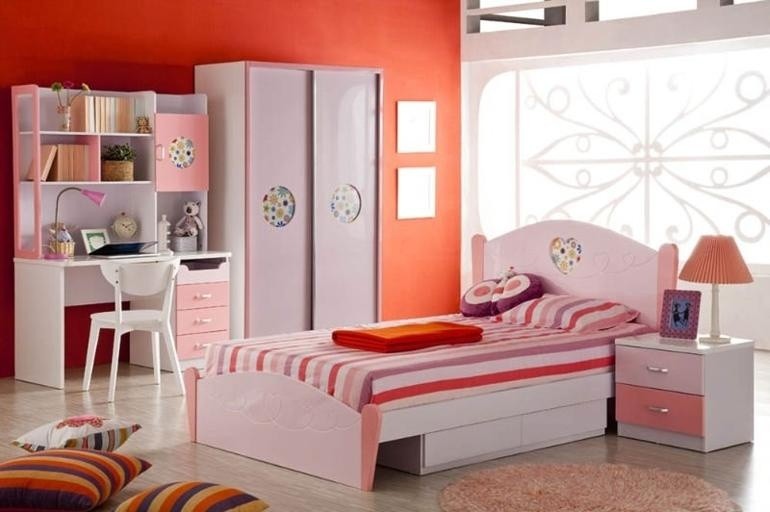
[673,301,691,329]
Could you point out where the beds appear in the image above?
[183,219,680,491]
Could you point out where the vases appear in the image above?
[55,106,70,131]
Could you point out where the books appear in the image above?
[70,95,135,133]
[28,143,90,182]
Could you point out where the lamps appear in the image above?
[678,235,754,344]
[44,187,106,260]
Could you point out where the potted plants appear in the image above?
[101,142,137,181]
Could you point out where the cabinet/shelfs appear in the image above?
[194,60,384,339]
[614,333,754,454]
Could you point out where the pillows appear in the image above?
[0,448,152,512]
[489,293,641,336]
[461,267,543,317]
[108,480,270,512]
[10,415,142,453]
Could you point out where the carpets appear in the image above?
[437,462,743,512]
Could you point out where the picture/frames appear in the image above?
[659,289,701,339]
[80,229,110,255]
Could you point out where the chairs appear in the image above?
[81,256,186,402]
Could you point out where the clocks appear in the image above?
[111,211,138,238]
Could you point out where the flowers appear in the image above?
[52,81,89,108]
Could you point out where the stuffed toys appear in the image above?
[174,199,204,236]
[49,222,72,244]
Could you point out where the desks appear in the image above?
[11,84,232,390]
